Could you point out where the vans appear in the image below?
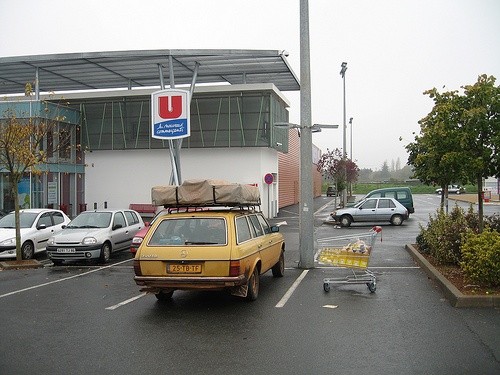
[345,187,415,217]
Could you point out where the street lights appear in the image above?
[348,117,354,195]
[339,61,349,208]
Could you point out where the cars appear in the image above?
[130,208,214,259]
[326,186,338,197]
[435,184,465,195]
[45,210,145,264]
[0,208,71,260]
[330,197,409,227]
[133,204,285,301]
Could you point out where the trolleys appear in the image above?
[316,226,382,293]
